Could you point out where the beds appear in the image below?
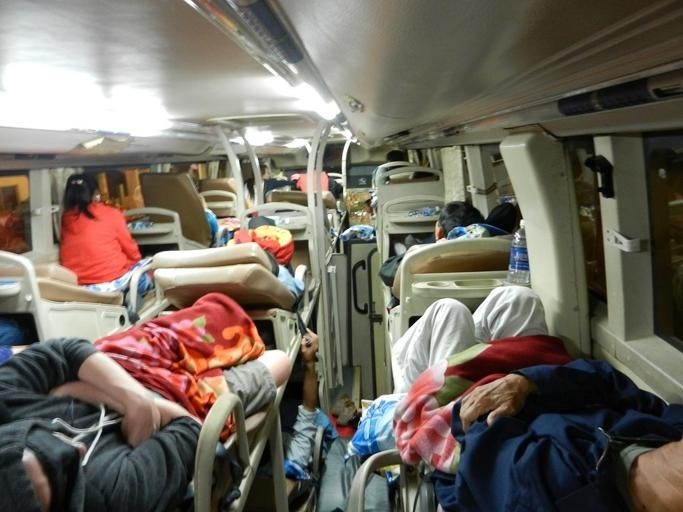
[348,160,531,512]
[0,173,340,512]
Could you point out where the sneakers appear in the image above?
[332,393,356,425]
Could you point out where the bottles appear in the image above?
[507,220,529,282]
[408,205,439,218]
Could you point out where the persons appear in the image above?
[283,322,326,486]
[59,171,153,298]
[0,292,290,510]
[392,283,683,510]
[0,313,34,367]
